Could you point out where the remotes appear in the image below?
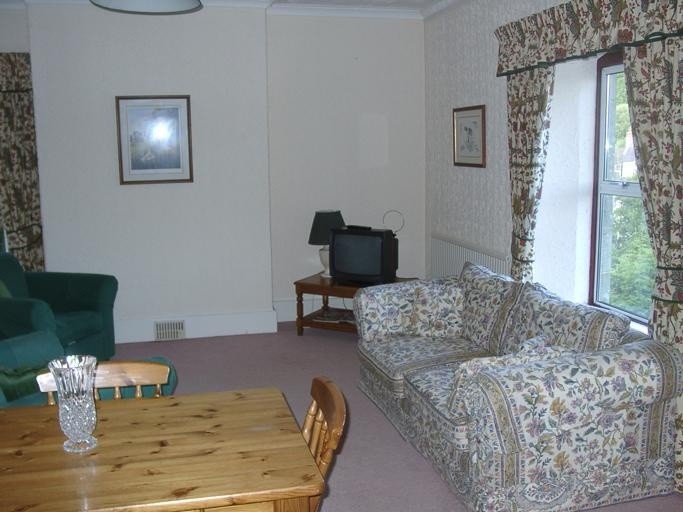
[347,224,371,230]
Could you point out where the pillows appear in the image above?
[407,287,465,339]
[444,334,571,421]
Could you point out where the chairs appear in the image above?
[300,376,348,480]
[0,223,177,408]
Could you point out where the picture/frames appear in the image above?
[451,103,486,168]
[114,94,193,185]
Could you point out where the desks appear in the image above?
[292,270,419,337]
[0,387,325,512]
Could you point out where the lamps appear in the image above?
[307,210,346,279]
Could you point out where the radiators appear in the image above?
[429,231,511,286]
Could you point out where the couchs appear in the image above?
[351,261,682,512]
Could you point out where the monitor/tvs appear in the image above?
[329,227,398,289]
